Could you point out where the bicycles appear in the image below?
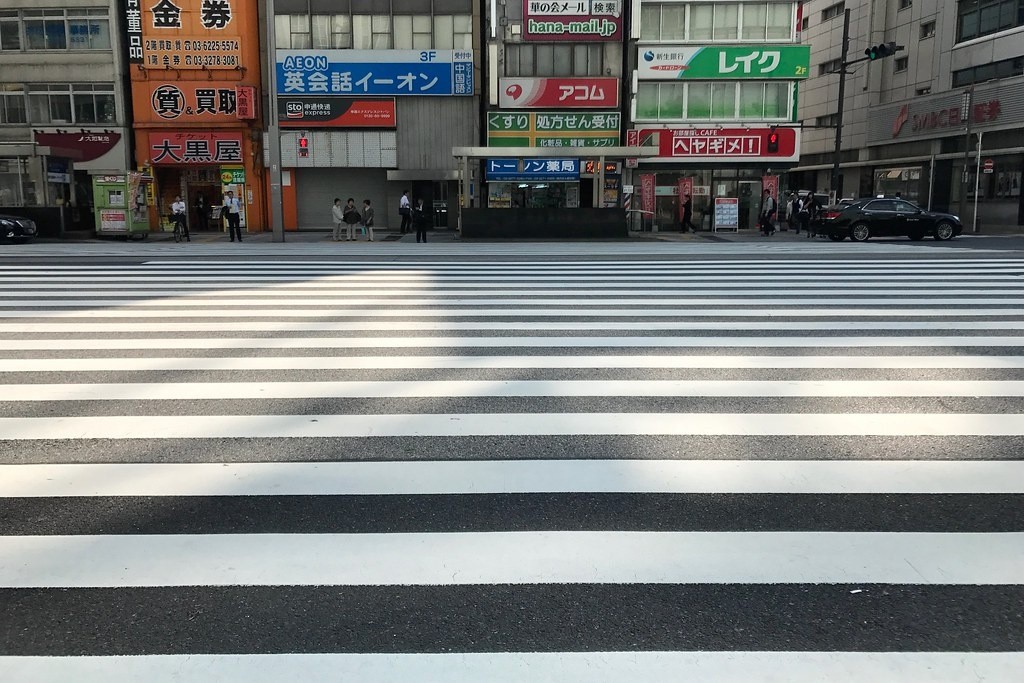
[174,209,185,243]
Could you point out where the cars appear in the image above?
[813,197,963,242]
[0,214,38,245]
[782,189,855,224]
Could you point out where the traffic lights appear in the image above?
[298,131,310,158]
[768,128,778,153]
[865,42,896,61]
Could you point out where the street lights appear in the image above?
[961,82,974,233]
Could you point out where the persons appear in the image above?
[760,189,777,238]
[225,191,243,242]
[344,198,360,241]
[361,199,375,241]
[194,191,211,230]
[172,194,191,243]
[786,191,823,238]
[894,192,903,211]
[413,197,428,243]
[332,197,345,241]
[680,194,696,234]
[399,189,413,233]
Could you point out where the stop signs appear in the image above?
[984,160,994,174]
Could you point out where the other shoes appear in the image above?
[400,228,413,233]
[368,239,374,242]
[346,238,357,241]
[187,236,190,242]
[239,239,242,242]
[230,239,234,242]
[417,240,427,243]
[333,238,342,241]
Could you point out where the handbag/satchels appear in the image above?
[362,226,366,236]
[399,204,410,215]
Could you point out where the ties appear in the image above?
[231,198,232,205]
